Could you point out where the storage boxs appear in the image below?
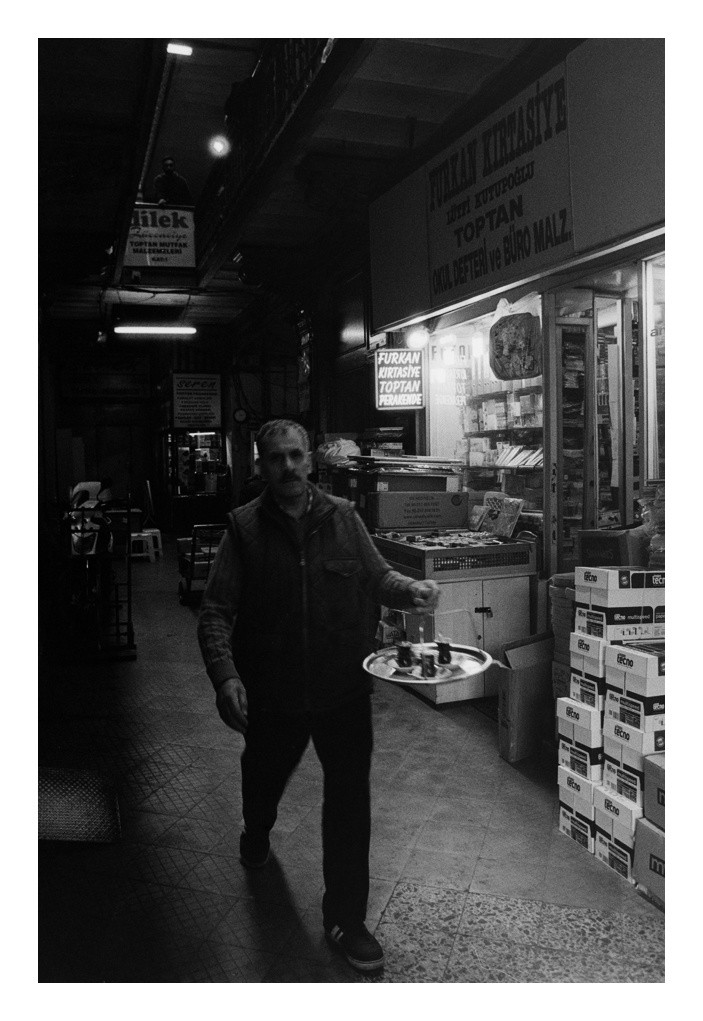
[178,546,220,578]
[492,564,665,912]
[366,491,470,528]
[505,471,543,510]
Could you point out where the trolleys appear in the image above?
[175,523,229,608]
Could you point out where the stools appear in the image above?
[123,529,164,569]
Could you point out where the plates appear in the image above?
[387,651,464,680]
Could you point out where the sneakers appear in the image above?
[240,821,272,869]
[323,921,386,972]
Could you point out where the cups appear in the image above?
[420,653,437,677]
[395,640,412,666]
[434,637,452,663]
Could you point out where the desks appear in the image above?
[105,509,143,553]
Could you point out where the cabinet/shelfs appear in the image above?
[406,577,531,710]
[462,385,543,477]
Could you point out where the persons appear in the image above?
[197,419,441,969]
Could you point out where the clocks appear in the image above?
[233,409,250,423]
